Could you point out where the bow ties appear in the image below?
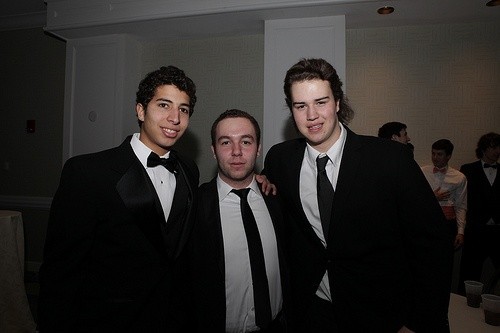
[483,163,498,169]
[433,167,447,173]
[147,150,179,175]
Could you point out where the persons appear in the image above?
[420,138,468,292]
[39,65,276,333]
[185,108,301,333]
[378,121,451,203]
[459,132,500,301]
[260,57,451,333]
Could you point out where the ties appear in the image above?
[230,188,274,330]
[315,155,337,246]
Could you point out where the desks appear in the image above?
[0,211,24,278]
[447,293,500,333]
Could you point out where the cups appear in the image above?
[481,294,500,326]
[464,281,483,308]
